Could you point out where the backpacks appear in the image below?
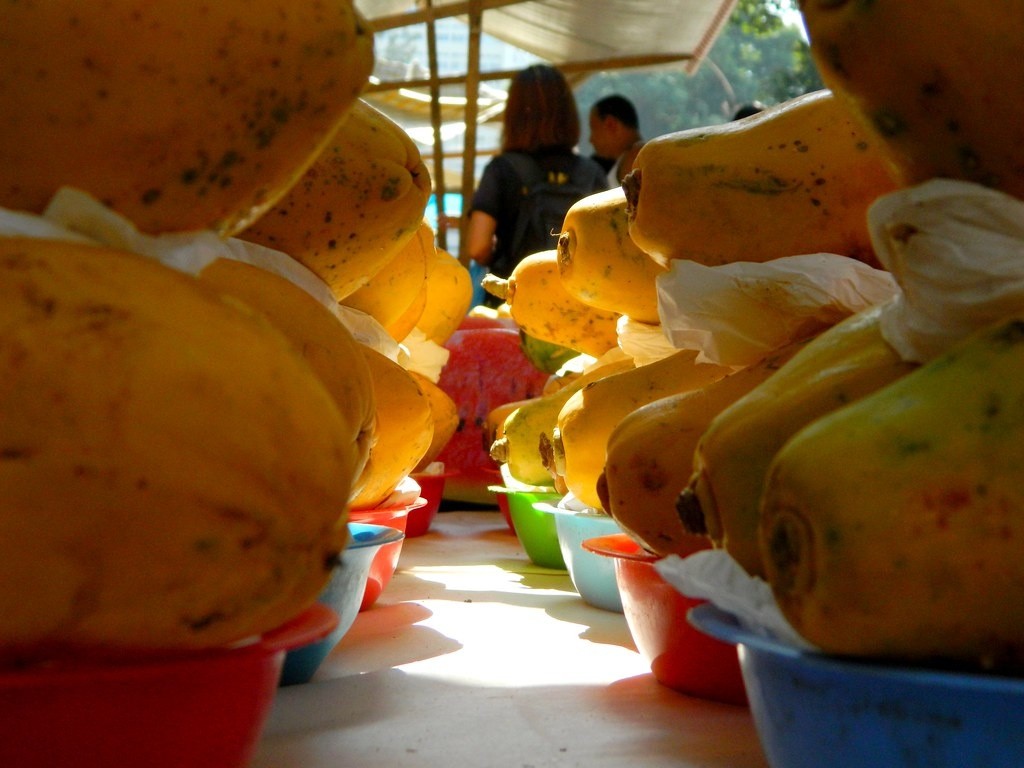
[502,150,598,261]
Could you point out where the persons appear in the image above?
[468,64,612,306]
[586,94,646,190]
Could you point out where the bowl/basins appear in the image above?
[478,455,1024,767]
[1,459,472,768]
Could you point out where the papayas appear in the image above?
[0,0,1024,676]
